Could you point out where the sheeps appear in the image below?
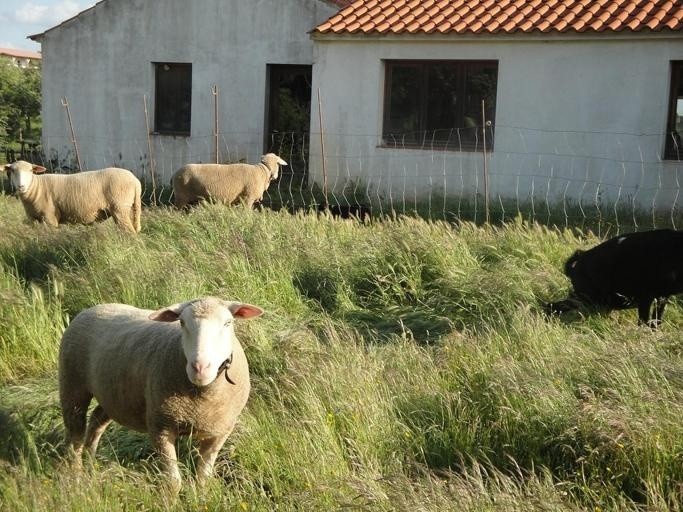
[0,160,141,234]
[57,296,264,511]
[564,227,682,331]
[169,152,288,215]
